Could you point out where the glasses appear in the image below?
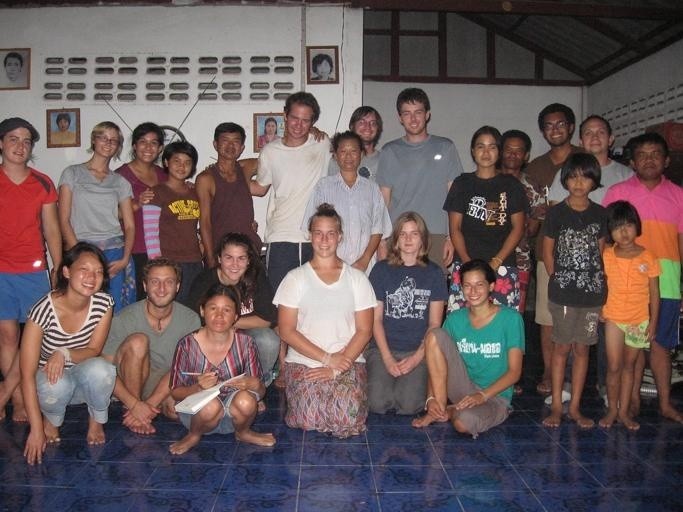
[95,134,118,145]
[543,120,570,130]
[358,118,377,126]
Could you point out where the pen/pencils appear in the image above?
[181,372,217,376]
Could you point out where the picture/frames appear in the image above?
[46,108,80,148]
[306,46,339,84]
[0,48,31,90]
[253,113,285,153]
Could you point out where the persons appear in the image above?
[310,54,335,82]
[1,51,27,88]
[0,88,683,465]
